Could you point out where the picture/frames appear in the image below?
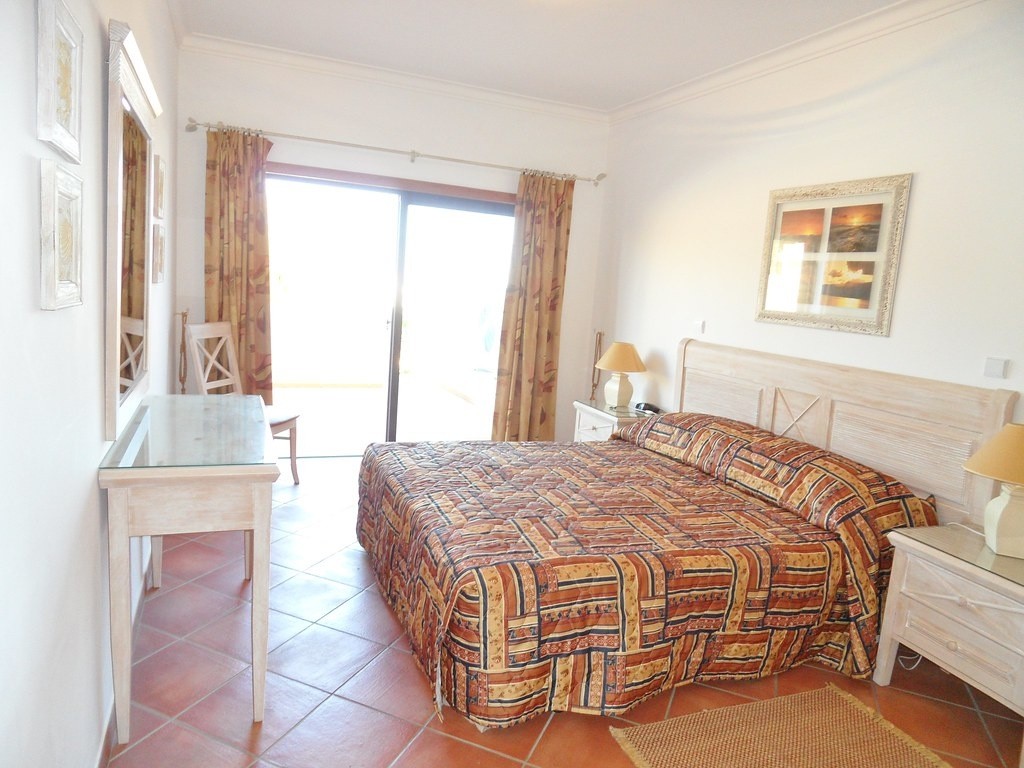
[37,0,85,163]
[155,156,166,219]
[755,173,912,339]
[41,160,85,310]
[152,224,165,284]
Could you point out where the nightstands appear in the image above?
[871,523,1024,768]
[573,400,651,441]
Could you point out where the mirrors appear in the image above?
[105,20,164,441]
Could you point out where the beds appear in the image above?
[357,339,1020,732]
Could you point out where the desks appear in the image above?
[97,395,281,745]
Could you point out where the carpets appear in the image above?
[609,680,951,768]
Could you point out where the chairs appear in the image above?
[185,320,299,484]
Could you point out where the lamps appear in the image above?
[595,341,646,405]
[962,423,1024,560]
